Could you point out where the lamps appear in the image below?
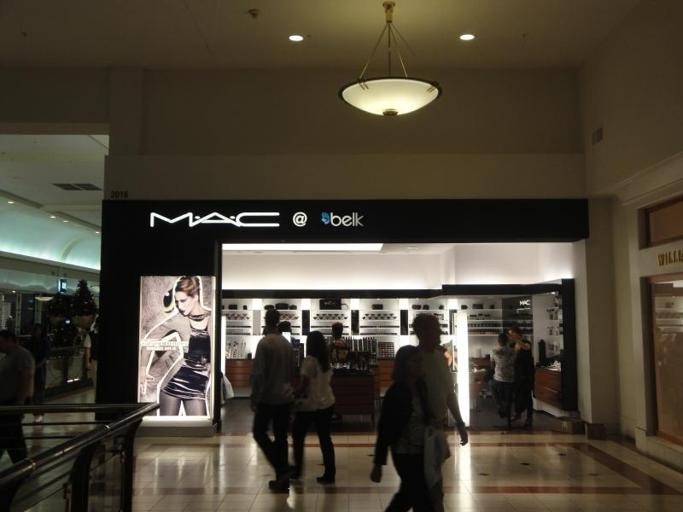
[338,2,442,116]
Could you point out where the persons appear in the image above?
[250,309,349,491]
[370,313,468,512]
[139,276,212,416]
[23,323,50,423]
[0,329,36,481]
[490,326,535,428]
[5,316,16,334]
[83,316,100,402]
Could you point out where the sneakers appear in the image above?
[269,479,289,488]
[316,472,335,483]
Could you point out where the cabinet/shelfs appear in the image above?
[227,360,254,388]
[359,310,400,336]
[470,357,490,405]
[222,309,253,335]
[469,309,503,335]
[311,309,351,337]
[533,368,562,406]
[408,310,450,336]
[261,310,302,336]
[333,376,375,430]
[379,359,396,394]
[544,307,561,337]
